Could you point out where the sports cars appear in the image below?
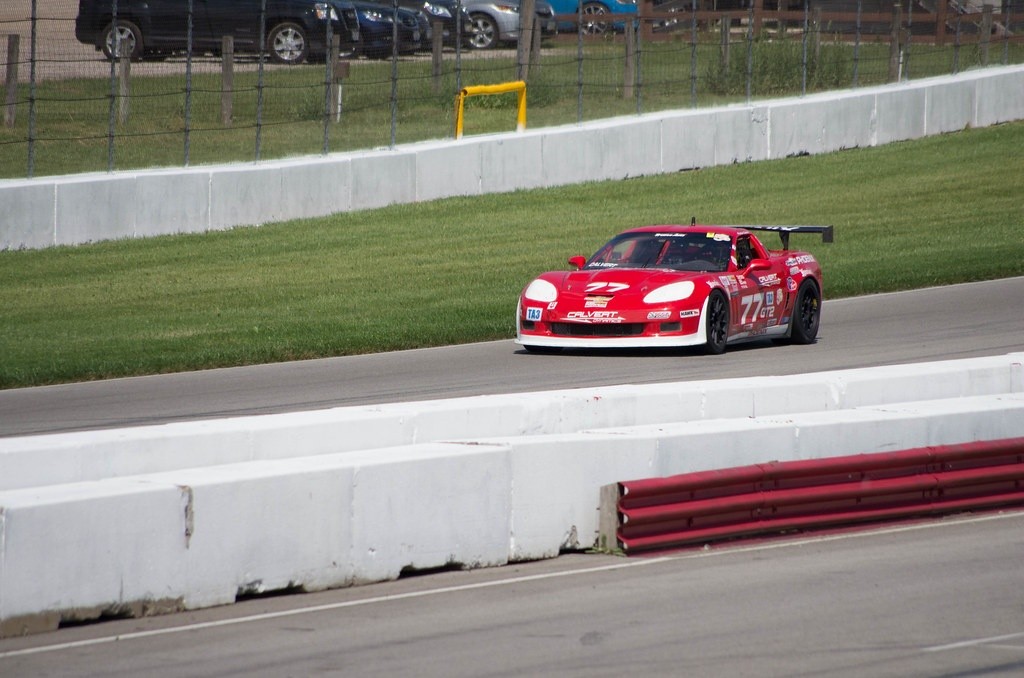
[514,216,834,354]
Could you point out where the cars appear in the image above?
[376,0,696,50]
[74,0,429,65]
